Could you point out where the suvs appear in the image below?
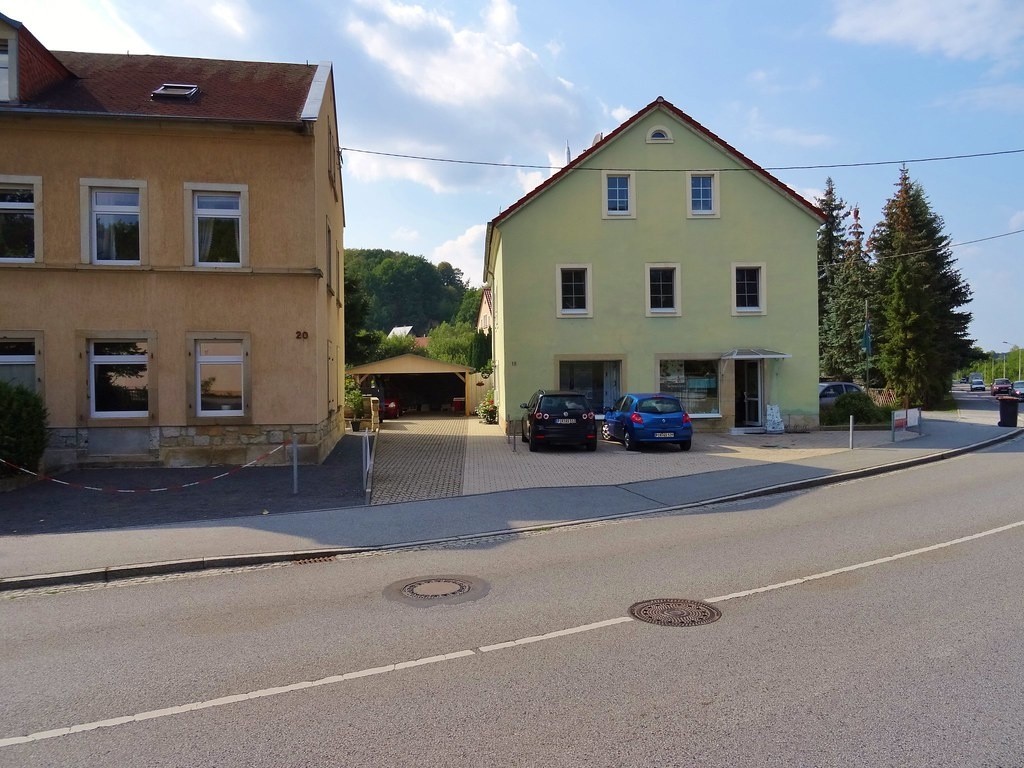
[519,389,598,452]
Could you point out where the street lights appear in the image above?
[1002,342,1020,381]
[989,357,993,380]
[999,353,1005,378]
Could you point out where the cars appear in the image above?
[1008,381,1024,400]
[960,378,967,383]
[971,380,985,392]
[378,388,408,420]
[991,378,1013,395]
[818,383,865,406]
[601,393,694,450]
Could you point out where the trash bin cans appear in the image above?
[996,396,1022,428]
